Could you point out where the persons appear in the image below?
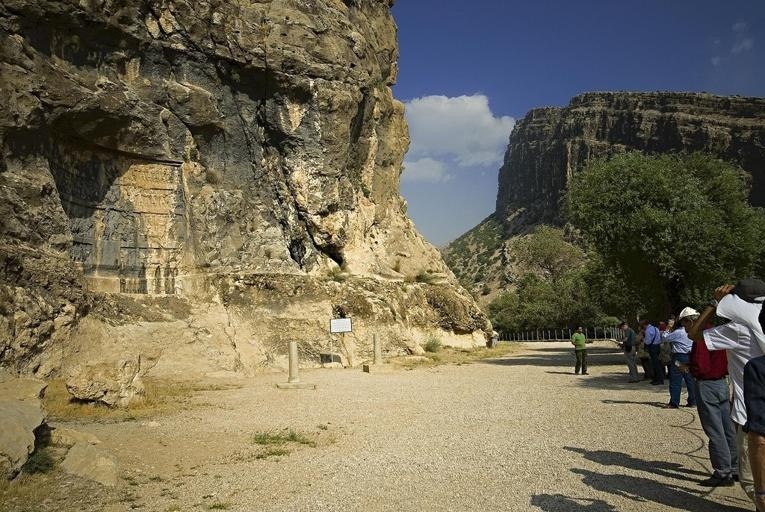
[571,326,589,375]
[610,279,765,512]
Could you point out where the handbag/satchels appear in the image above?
[625,345,632,352]
[639,344,649,360]
[644,343,652,352]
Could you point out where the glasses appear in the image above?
[619,326,622,329]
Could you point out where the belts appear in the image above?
[697,377,726,380]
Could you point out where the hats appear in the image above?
[665,313,675,322]
[616,321,627,327]
[676,306,701,324]
[729,280,765,303]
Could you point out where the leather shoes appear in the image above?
[650,381,664,385]
[686,404,696,407]
[662,403,678,408]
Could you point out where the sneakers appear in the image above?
[582,373,589,375]
[629,380,640,383]
[575,372,580,374]
[704,471,739,486]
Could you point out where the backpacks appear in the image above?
[660,343,671,365]
